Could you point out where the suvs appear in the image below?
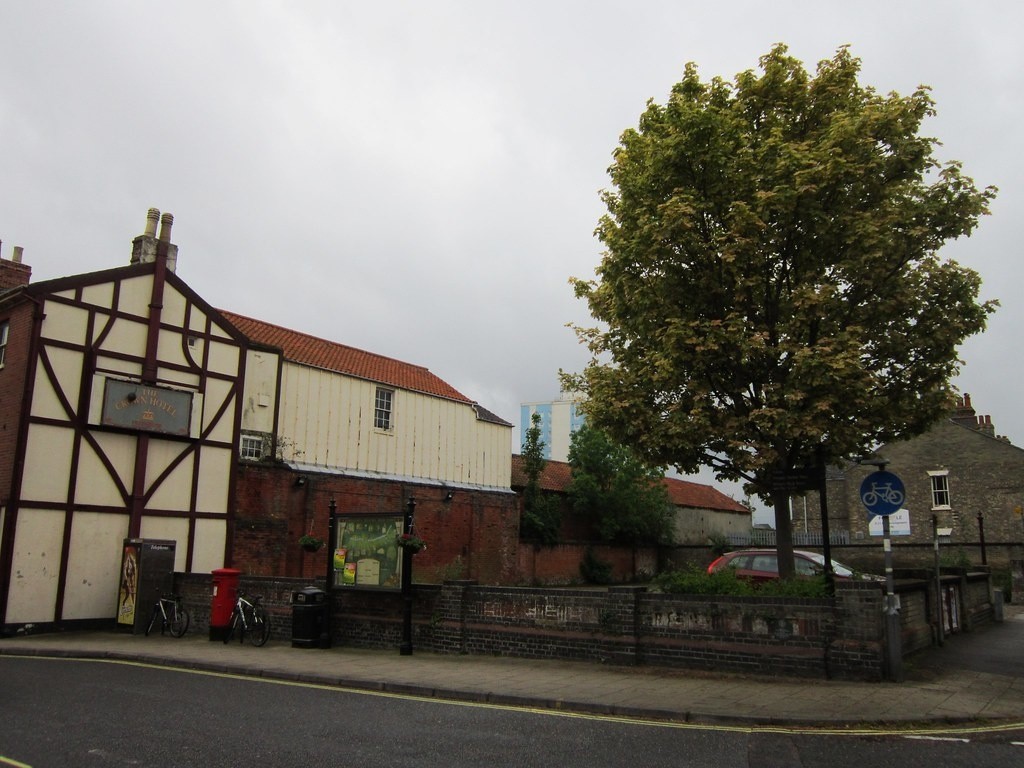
[708,549,886,595]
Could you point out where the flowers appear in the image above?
[394,534,426,549]
[298,535,326,549]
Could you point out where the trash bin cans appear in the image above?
[291,585,331,649]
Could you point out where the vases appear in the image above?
[303,545,319,553]
[404,546,421,554]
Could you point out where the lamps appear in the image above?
[292,474,307,489]
[443,491,455,502]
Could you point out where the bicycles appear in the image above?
[222,586,271,647]
[146,588,190,638]
